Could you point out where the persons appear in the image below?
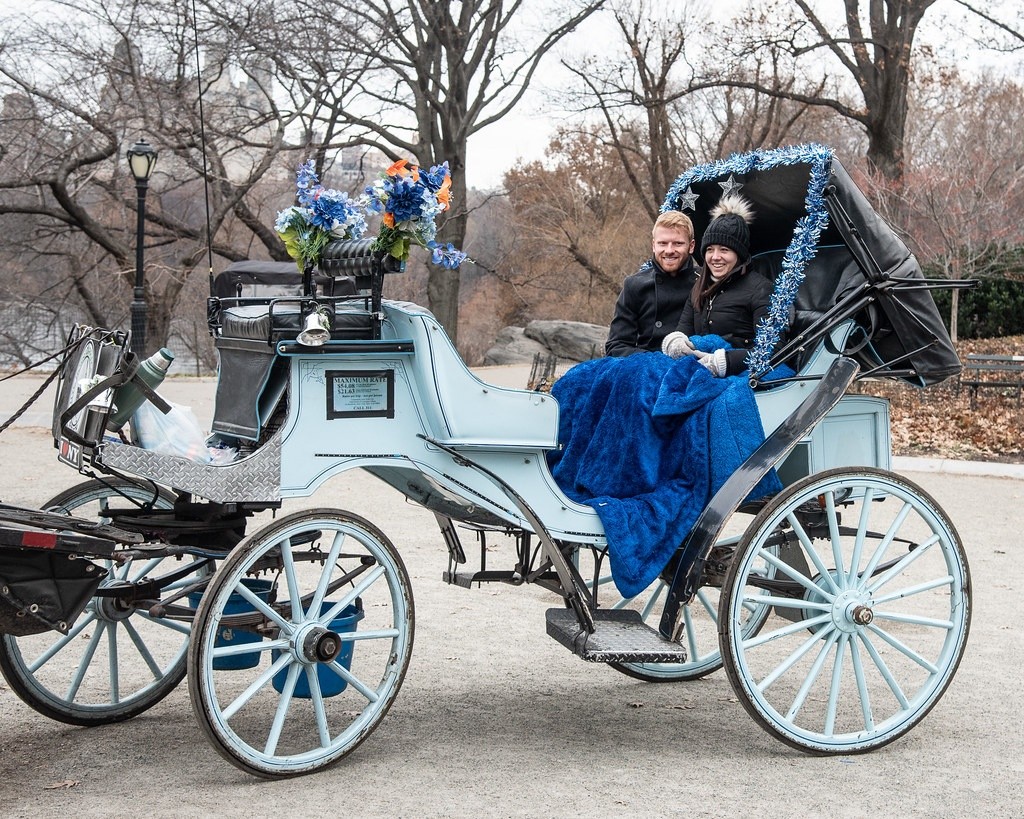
[661,212,791,379]
[604,211,705,366]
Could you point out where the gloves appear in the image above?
[661,331,695,361]
[693,348,727,378]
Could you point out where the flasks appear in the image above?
[105,347,175,433]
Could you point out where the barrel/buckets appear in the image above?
[185,578,277,669]
[268,547,364,698]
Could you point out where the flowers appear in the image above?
[359,157,480,270]
[274,157,367,276]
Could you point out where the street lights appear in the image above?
[126,138,158,360]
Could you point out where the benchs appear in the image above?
[211,260,565,451]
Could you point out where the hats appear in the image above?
[700,195,754,262]
